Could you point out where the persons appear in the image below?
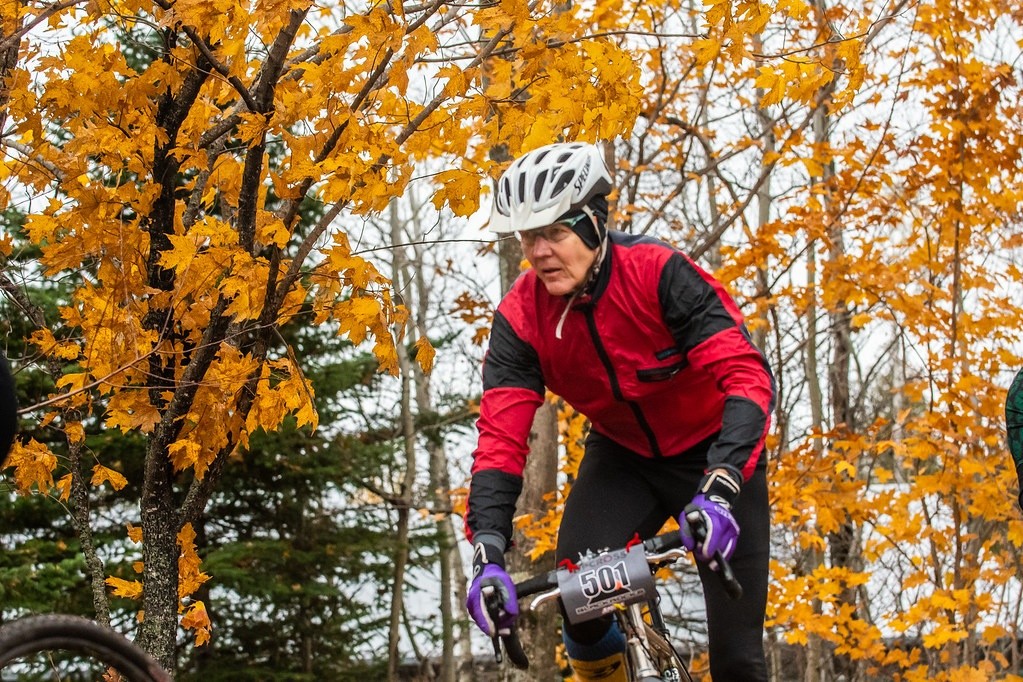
[464,143,777,682]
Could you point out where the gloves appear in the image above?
[679,472,740,573]
[467,532,518,639]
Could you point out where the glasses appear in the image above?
[513,207,595,246]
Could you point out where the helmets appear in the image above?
[490,143,612,233]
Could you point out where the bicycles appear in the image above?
[486,502,745,682]
[0,613,176,682]
